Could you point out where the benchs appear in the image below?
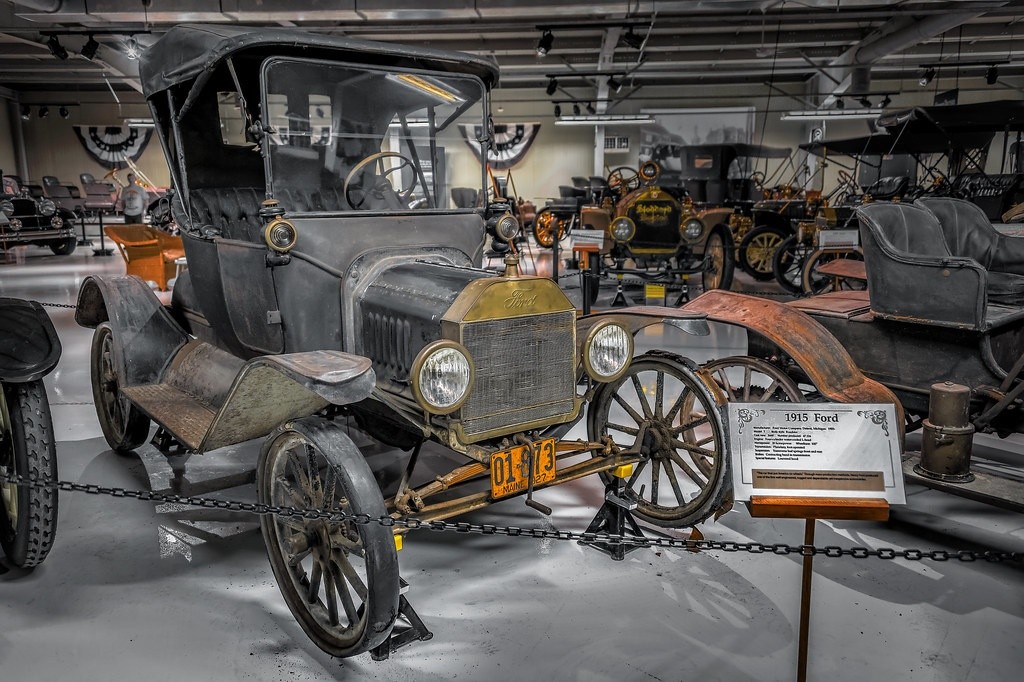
[845,175,911,205]
[948,173,1019,221]
[188,168,403,245]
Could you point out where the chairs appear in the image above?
[558,185,586,199]
[572,176,590,187]
[589,176,608,187]
[854,202,1024,334]
[4,174,116,198]
[103,221,186,293]
[450,187,477,209]
[912,194,1024,306]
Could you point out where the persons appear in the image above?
[121,173,150,224]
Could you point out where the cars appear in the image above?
[533,99,1024,482]
[75,22,734,658]
[0,168,78,255]
[680,196,1024,540]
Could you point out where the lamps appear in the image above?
[38,106,49,120]
[553,116,656,125]
[124,35,140,60]
[80,34,100,61]
[20,106,33,121]
[836,97,845,110]
[59,106,70,120]
[554,103,561,117]
[860,97,872,108]
[986,65,999,85]
[918,68,937,88]
[118,115,155,128]
[572,103,581,116]
[778,110,884,122]
[623,27,644,50]
[546,77,559,95]
[606,76,622,94]
[536,30,555,58]
[46,36,69,61]
[586,103,597,115]
[388,117,438,127]
[879,95,891,109]
